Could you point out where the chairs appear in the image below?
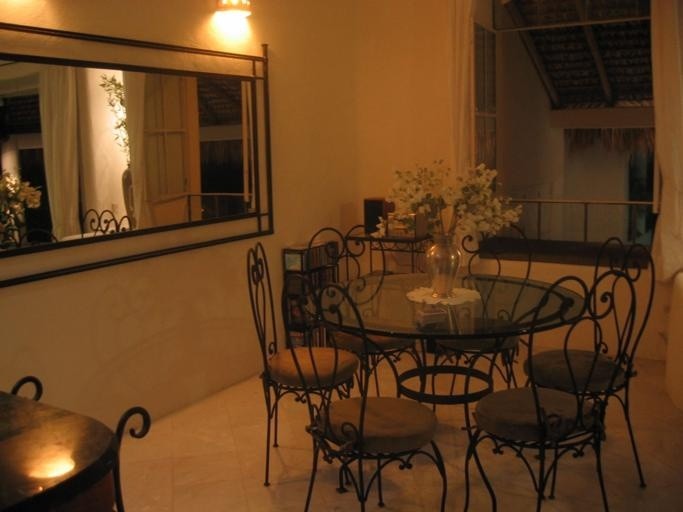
[82,209,132,238]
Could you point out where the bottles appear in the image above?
[416,206,428,237]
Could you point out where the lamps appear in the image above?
[217,0,252,16]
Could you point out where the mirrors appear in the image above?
[0,22,274,288]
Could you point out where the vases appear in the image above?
[121,164,136,230]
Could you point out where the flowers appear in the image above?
[389,160,524,237]
[0,169,42,244]
[101,76,130,165]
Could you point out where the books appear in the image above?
[387,212,416,240]
[280,239,338,349]
